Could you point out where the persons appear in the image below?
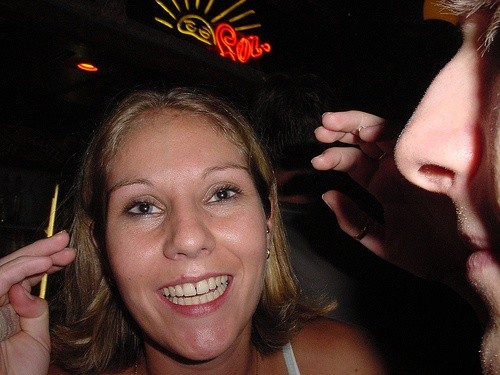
[241,69,425,375]
[308,0,500,375]
[0,83,393,375]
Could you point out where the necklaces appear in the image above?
[129,344,259,375]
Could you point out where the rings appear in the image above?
[373,153,390,162]
[348,219,373,243]
